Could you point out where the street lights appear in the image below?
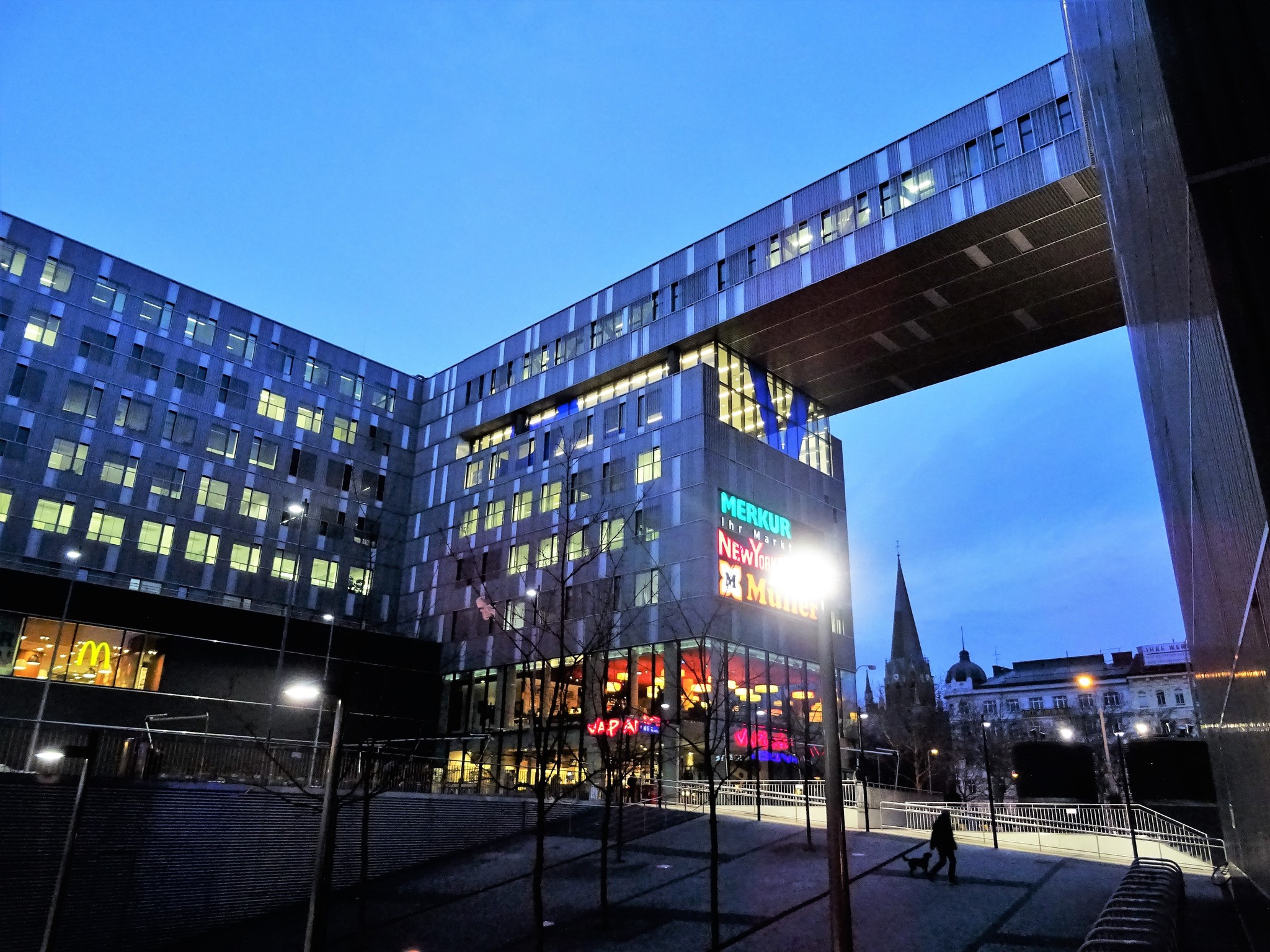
[251,498,307,784]
[19,545,87,772]
[34,741,101,952]
[781,541,857,952]
[300,613,337,787]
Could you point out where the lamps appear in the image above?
[606,667,825,723]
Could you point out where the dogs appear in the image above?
[898,852,932,879]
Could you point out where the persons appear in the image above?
[1106,787,1127,834]
[625,773,638,803]
[929,809,959,883]
